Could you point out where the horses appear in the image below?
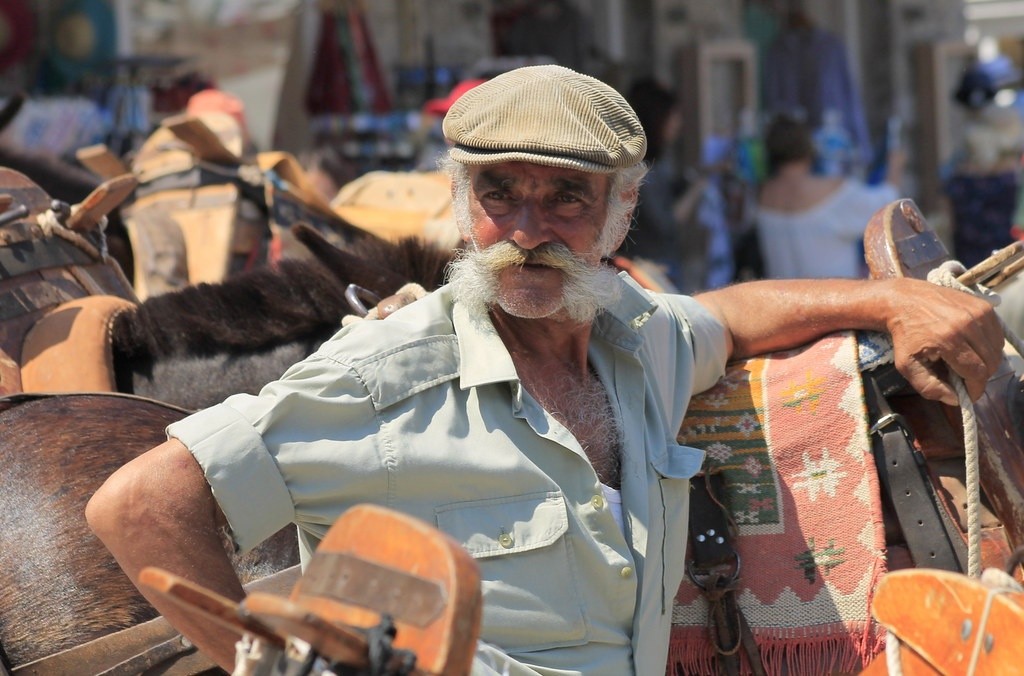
[0,233,1024,676]
[0,77,456,409]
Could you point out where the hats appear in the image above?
[441,64,648,176]
[185,86,257,149]
[423,79,489,118]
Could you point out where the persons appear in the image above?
[83,65,1006,675]
[954,71,1024,245]
[626,75,745,288]
[753,117,904,279]
